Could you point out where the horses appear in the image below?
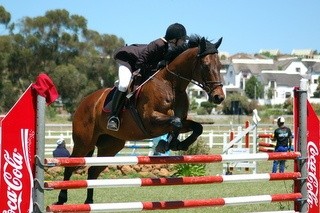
[57,34,225,204]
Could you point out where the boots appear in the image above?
[107,89,126,131]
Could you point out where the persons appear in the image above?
[153,134,171,156]
[108,23,189,128]
[270,116,293,173]
[52,138,70,158]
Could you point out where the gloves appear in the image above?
[157,60,166,70]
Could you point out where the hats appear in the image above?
[57,139,64,144]
[274,117,285,123]
[166,23,190,39]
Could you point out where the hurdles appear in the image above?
[33,74,309,213]
